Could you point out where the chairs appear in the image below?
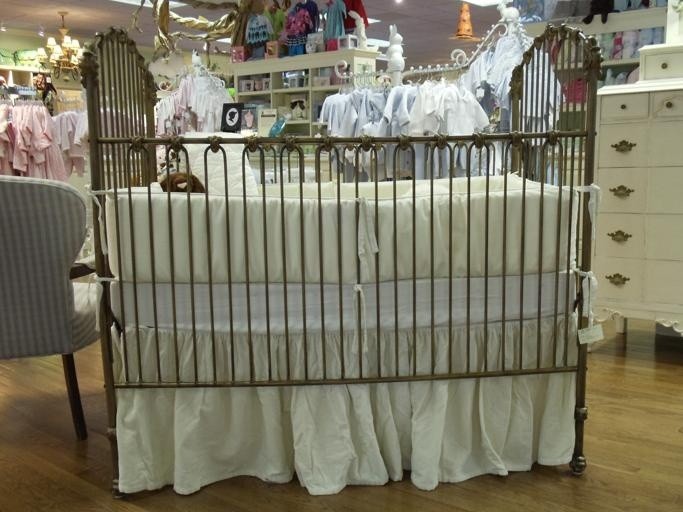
[0,172,102,441]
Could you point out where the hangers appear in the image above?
[163,64,227,95]
[340,8,537,94]
[1,93,94,114]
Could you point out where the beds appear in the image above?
[77,21,605,501]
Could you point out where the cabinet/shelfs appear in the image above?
[549,0,683,156]
[0,65,53,94]
[234,25,391,123]
[590,34,683,355]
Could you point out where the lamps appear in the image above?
[37,12,87,83]
